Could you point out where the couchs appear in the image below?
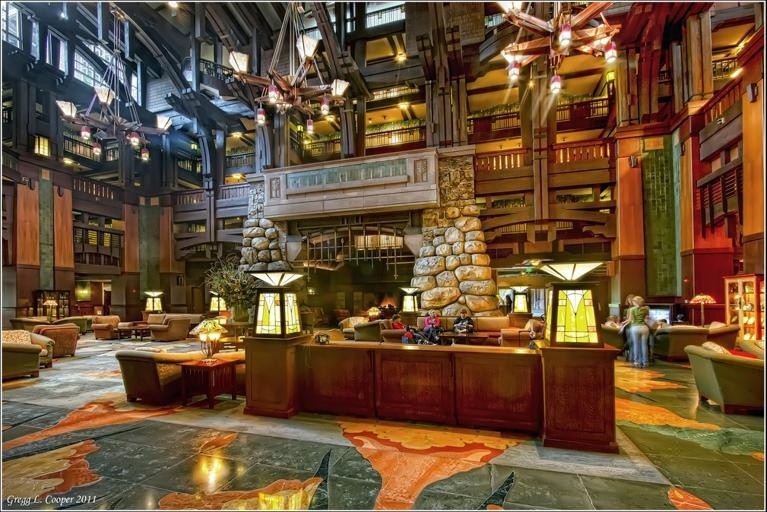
[416,316,509,346]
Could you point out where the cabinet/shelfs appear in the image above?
[723,274,764,341]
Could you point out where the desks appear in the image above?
[438,331,489,345]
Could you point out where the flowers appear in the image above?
[197,253,256,309]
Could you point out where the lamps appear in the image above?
[208,288,227,311]
[143,290,164,311]
[228,1,349,135]
[689,293,716,328]
[398,286,421,312]
[510,256,605,349]
[56,6,173,162]
[42,299,58,316]
[501,2,622,96]
[244,268,306,338]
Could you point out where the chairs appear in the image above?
[601,321,765,414]
[3,311,246,408]
[500,318,543,347]
[338,304,413,343]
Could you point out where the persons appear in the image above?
[621,294,635,362]
[618,296,651,369]
[392,314,438,346]
[423,309,445,342]
[452,309,475,333]
[505,295,513,314]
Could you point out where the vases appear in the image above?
[233,305,249,321]
